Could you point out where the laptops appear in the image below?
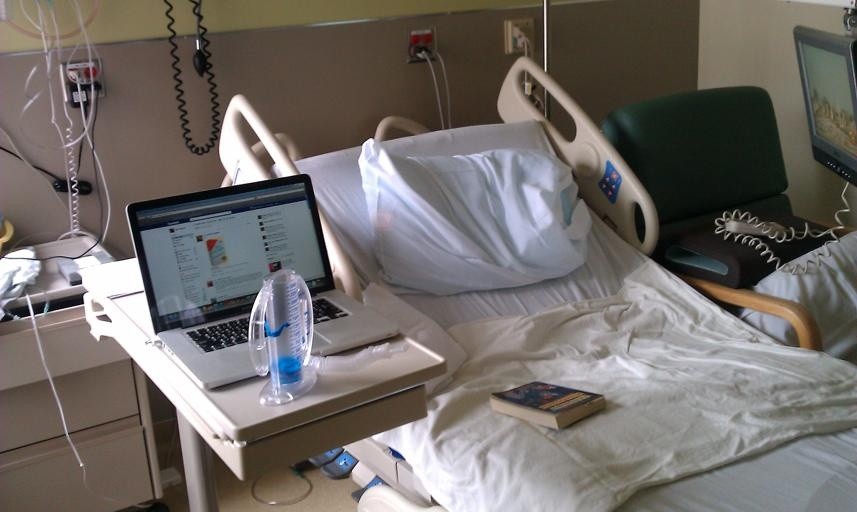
[126,174,402,389]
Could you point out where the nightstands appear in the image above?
[2,234,162,512]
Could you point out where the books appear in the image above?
[488,380,607,431]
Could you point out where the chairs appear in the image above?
[597,84,846,284]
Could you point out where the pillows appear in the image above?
[355,133,593,296]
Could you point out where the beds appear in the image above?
[215,55,857,510]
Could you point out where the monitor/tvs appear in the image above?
[793,25,857,187]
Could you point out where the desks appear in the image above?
[76,252,447,512]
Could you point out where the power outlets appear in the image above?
[502,16,537,57]
